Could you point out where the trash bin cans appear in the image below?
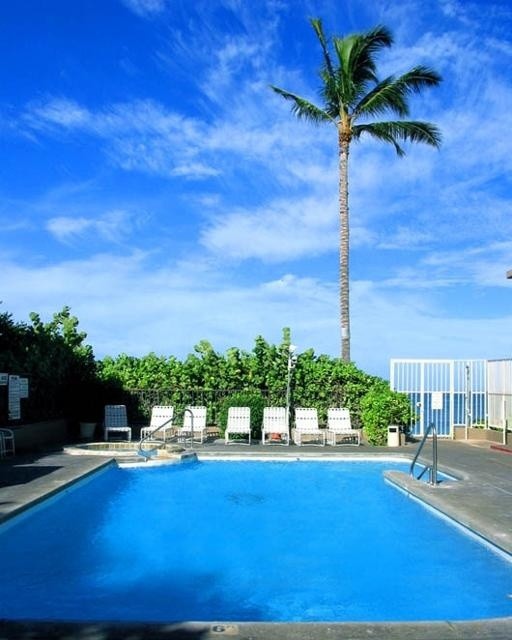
[387,426,399,447]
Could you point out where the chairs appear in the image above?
[102,404,361,448]
